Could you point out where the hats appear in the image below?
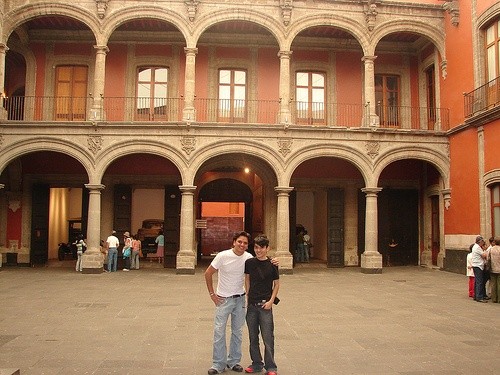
[124,232,130,237]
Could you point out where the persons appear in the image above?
[294,224,312,263]
[466,235,500,303]
[155,231,164,263]
[75,234,87,271]
[99,229,145,273]
[204,231,280,375]
[386,238,400,267]
[244,234,280,375]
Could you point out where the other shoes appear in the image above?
[484,296,491,300]
[478,299,488,303]
[123,268,129,271]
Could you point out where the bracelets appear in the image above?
[270,299,273,303]
[209,291,215,296]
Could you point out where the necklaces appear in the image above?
[233,249,244,256]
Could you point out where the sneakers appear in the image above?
[226,363,243,372]
[208,368,225,375]
[267,370,277,375]
[245,367,255,372]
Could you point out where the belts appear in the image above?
[218,292,246,298]
[248,299,268,303]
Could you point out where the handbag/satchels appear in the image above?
[308,243,312,248]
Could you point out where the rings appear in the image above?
[277,262,279,264]
